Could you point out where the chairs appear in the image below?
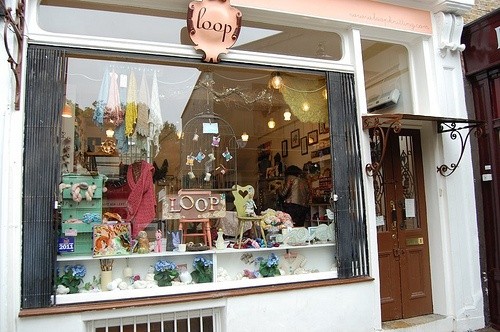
[232,184,267,249]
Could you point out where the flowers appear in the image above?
[260,208,295,231]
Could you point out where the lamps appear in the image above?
[62,104,72,117]
[106,127,114,138]
[268,112,276,128]
[283,109,291,120]
[242,131,249,141]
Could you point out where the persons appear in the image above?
[278,165,309,227]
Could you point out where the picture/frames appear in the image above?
[308,129,318,145]
[266,166,278,178]
[301,136,308,155]
[319,122,328,134]
[291,129,300,148]
[282,140,287,157]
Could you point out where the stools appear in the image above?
[179,218,212,250]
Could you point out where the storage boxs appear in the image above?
[61,173,102,233]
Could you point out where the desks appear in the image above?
[215,211,252,241]
[85,152,116,183]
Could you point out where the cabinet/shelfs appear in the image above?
[59,83,89,173]
[259,177,285,213]
[305,203,337,230]
[50,238,367,305]
